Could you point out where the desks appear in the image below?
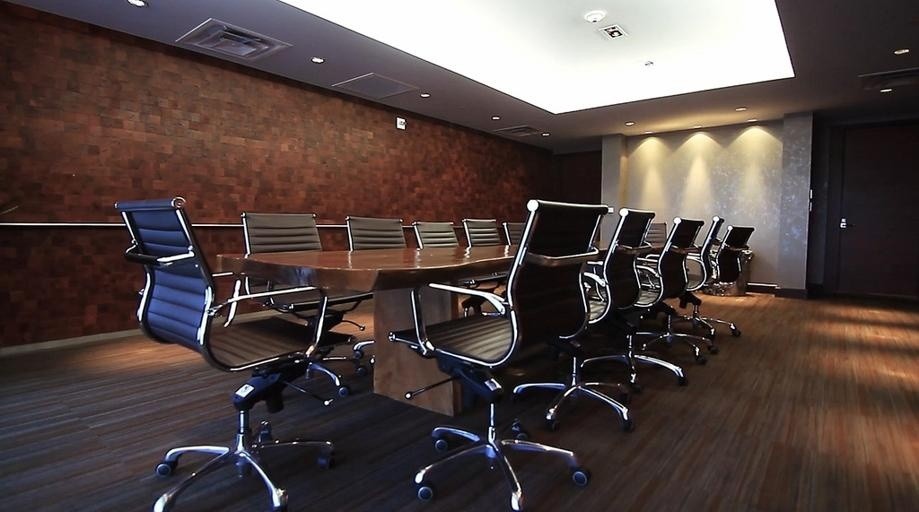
[212,243,704,419]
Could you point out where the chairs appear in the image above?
[113,198,334,512]
[236,208,367,401]
[386,200,753,510]
[341,215,410,250]
